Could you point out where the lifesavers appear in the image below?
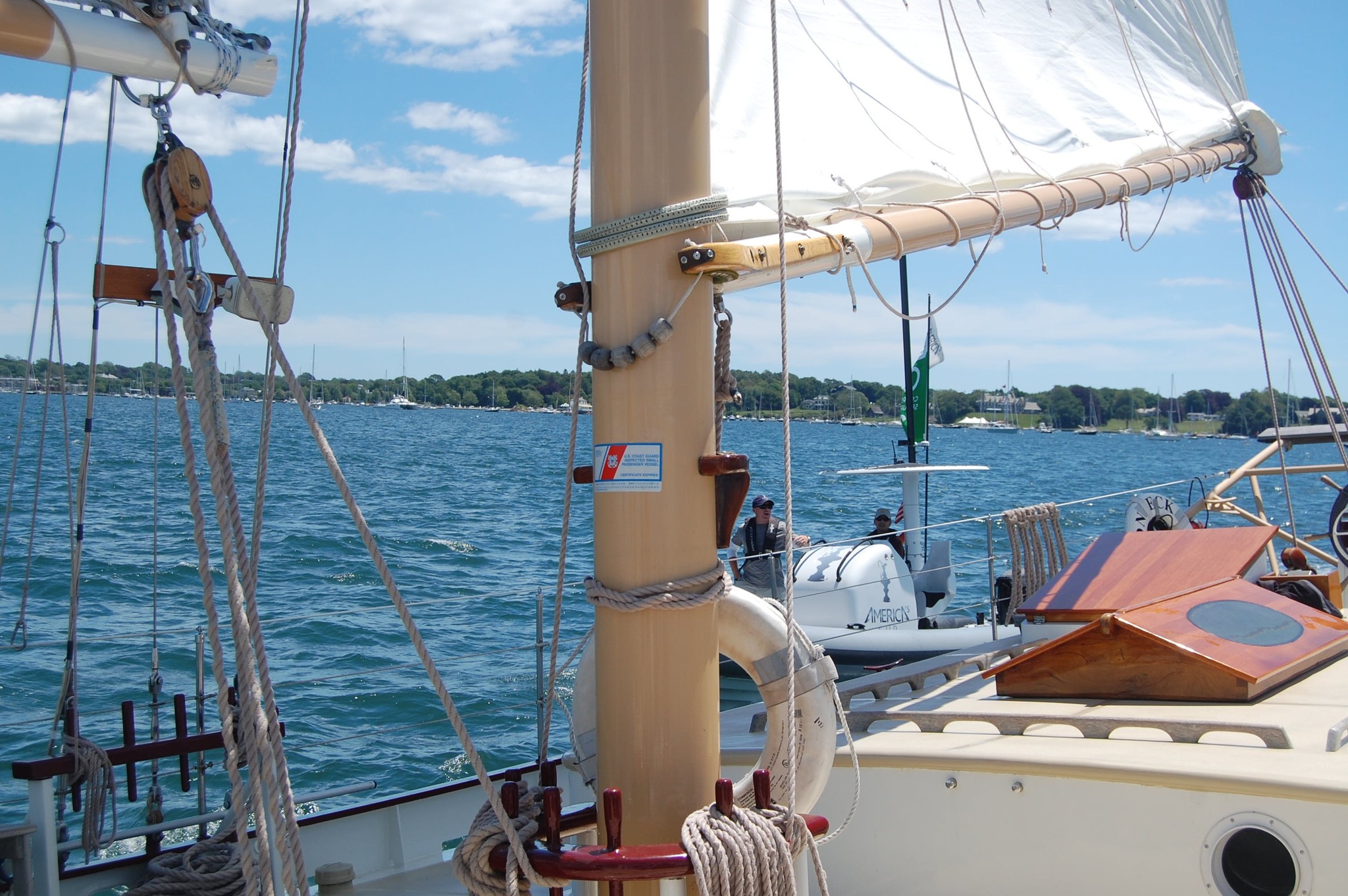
[1124,493,1192,531]
[570,587,837,817]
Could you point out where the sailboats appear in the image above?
[221,354,263,404]
[723,372,904,429]
[1073,388,1101,436]
[529,374,572,417]
[930,359,1062,435]
[1119,371,1262,442]
[75,369,153,401]
[484,379,501,413]
[0,361,47,395]
[272,336,445,411]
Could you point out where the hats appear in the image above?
[874,508,891,520]
[752,494,775,508]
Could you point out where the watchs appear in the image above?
[807,536,810,544]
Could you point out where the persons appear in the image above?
[867,508,897,540]
[727,495,812,599]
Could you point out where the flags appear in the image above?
[900,310,945,443]
[895,502,904,524]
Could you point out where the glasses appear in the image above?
[758,504,773,509]
[876,517,889,522]
